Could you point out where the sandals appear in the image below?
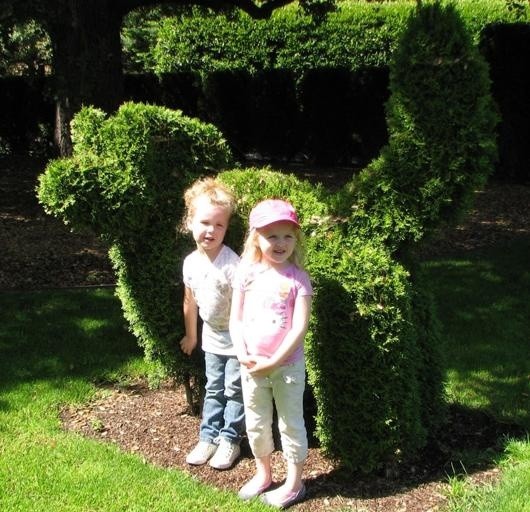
[239,477,306,508]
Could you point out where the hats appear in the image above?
[249,200,301,230]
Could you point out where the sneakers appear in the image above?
[186,442,240,469]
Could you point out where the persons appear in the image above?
[225,196,315,511]
[176,175,244,473]
